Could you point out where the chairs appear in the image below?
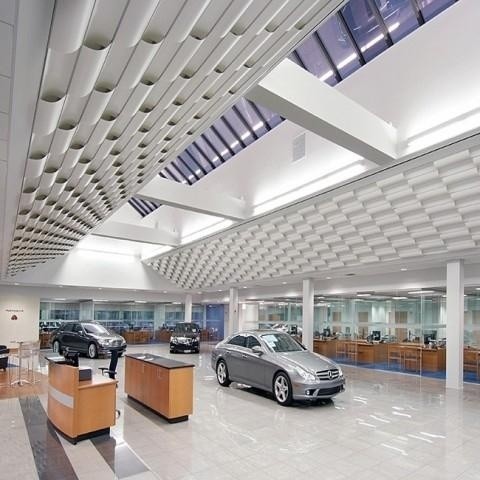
[100,344,126,419]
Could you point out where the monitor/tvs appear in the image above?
[64,350,79,366]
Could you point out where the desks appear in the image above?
[45,352,196,446]
[313,334,477,374]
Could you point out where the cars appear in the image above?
[48,320,127,357]
[212,330,345,405]
[170,321,201,353]
